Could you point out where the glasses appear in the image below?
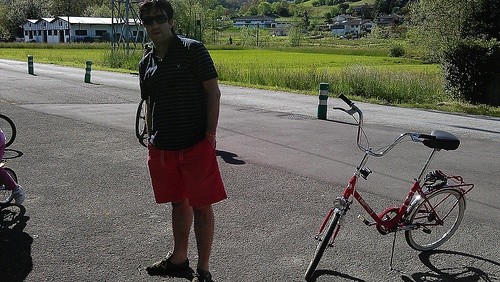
[141,14,170,27]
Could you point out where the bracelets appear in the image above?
[206,132,216,137]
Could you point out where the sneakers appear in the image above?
[145,253,189,273]
[192,269,212,282]
[13,185,25,205]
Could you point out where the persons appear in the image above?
[138,0,227,282]
[229,35,232,44]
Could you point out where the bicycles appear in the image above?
[0,113,19,208]
[304,92,475,281]
